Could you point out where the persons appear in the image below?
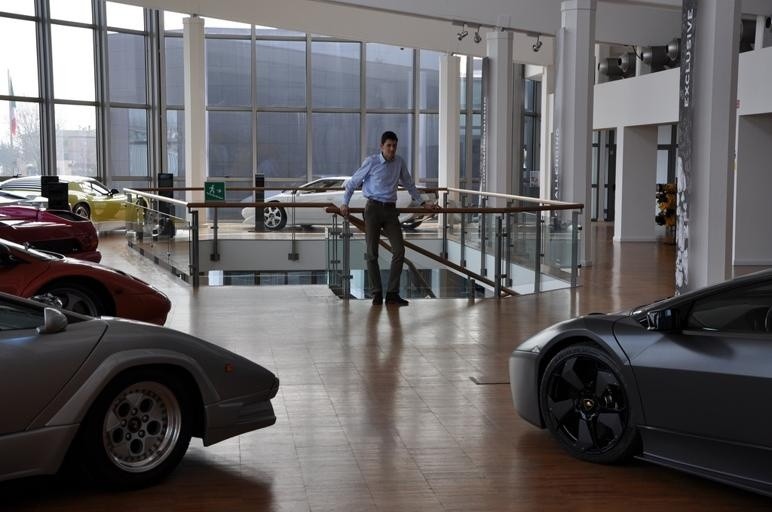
[208,184,215,195]
[339,131,442,306]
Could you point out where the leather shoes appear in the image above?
[373,293,383,305]
[386,292,408,304]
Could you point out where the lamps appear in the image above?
[532,33,542,52]
[457,24,468,40]
[473,25,482,43]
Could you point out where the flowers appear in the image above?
[654,183,678,227]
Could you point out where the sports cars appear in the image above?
[0,204,104,264]
[0,238,174,327]
[0,290,283,496]
[238,173,432,234]
[504,265,770,493]
[1,171,153,225]
